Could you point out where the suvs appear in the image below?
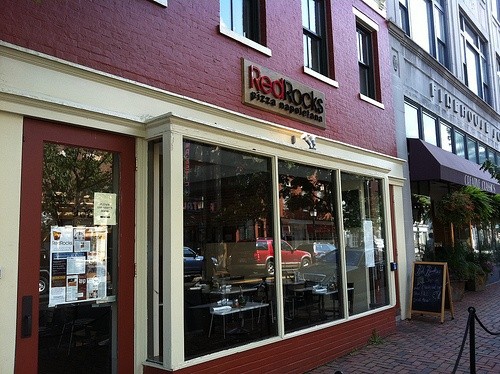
[230,238,312,280]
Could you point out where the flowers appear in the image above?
[448,271,466,281]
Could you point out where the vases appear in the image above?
[448,280,465,305]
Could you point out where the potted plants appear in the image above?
[461,262,486,291]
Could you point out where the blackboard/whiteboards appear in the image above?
[408,261,455,323]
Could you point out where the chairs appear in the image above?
[186,280,353,339]
[56,302,99,358]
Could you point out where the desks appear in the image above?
[202,287,257,333]
[266,280,316,319]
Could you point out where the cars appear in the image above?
[297,242,337,261]
[295,247,384,296]
[183,246,218,277]
